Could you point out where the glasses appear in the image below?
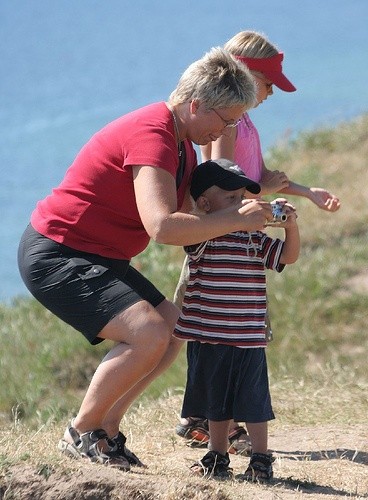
[211,109,243,128]
[256,75,272,89]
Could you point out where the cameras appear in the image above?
[265,203,287,225]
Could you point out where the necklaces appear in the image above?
[169,110,186,162]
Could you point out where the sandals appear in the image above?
[59,421,129,470]
[191,451,233,474]
[176,417,211,447]
[244,454,276,481]
[228,425,253,457]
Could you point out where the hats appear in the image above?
[190,158,261,201]
[235,52,297,91]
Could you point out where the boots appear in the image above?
[111,432,145,468]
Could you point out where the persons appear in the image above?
[175,31,341,458]
[171,158,300,484]
[16,48,298,473]
